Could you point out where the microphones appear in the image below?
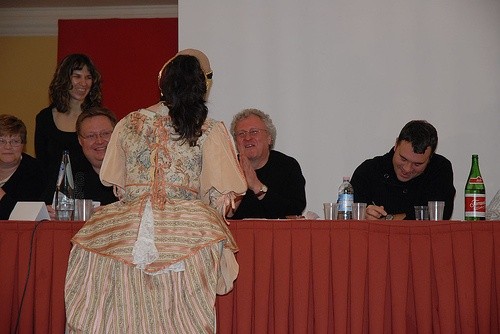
[53,149,71,220]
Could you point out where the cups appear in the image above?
[414,206,430,220]
[323,202,338,220]
[428,201,445,220]
[351,203,367,220]
[77,199,93,220]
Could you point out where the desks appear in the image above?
[0,220,500,334]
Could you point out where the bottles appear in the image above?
[52,148,76,219]
[337,176,355,219]
[464,154,486,220]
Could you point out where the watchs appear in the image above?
[255,184,267,198]
[385,214,394,221]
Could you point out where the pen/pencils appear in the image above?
[372,201,386,217]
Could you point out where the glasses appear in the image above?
[0,138,23,147]
[79,131,115,143]
[234,128,270,138]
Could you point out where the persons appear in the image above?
[0,114,50,221]
[63,48,248,334]
[33,53,103,205]
[47,107,121,218]
[222,109,307,220]
[336,119,456,221]
